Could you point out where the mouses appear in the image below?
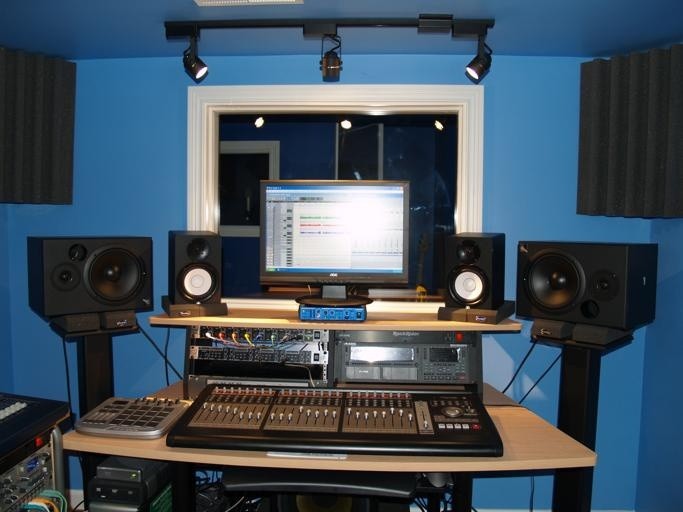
[427,472,447,488]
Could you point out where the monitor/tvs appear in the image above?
[258,179,409,311]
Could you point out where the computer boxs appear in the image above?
[88,485,174,512]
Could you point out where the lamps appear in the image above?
[464,19,492,84]
[319,18,343,82]
[182,19,209,82]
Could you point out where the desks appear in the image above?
[32,298,635,511]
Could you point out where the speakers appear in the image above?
[170,229,223,305]
[27,236,155,318]
[270,491,410,512]
[515,239,658,330]
[444,233,504,311]
